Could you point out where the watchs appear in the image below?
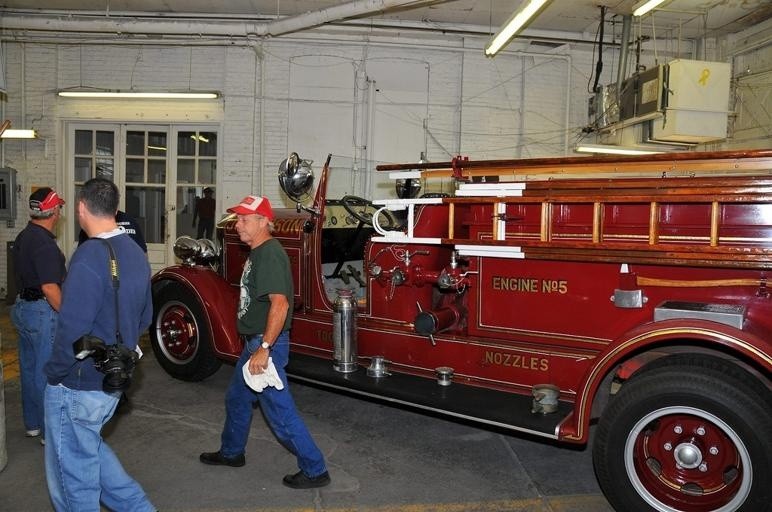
[259,339,274,352]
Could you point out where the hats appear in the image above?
[226,194,273,222]
[28,186,66,212]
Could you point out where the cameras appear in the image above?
[72,335,139,393]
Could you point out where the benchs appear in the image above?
[360,191,449,280]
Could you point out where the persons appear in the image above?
[200,196,335,487]
[70,192,149,406]
[126,186,142,224]
[190,187,219,239]
[8,188,98,443]
[43,178,154,512]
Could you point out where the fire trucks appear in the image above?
[149,144,771,512]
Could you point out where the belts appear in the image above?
[240,333,264,342]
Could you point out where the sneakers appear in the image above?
[199,449,246,468]
[25,426,45,446]
[282,468,331,490]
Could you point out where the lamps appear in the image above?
[54,42,223,105]
[482,1,552,60]
[1,126,44,143]
[630,1,667,19]
[575,139,673,156]
